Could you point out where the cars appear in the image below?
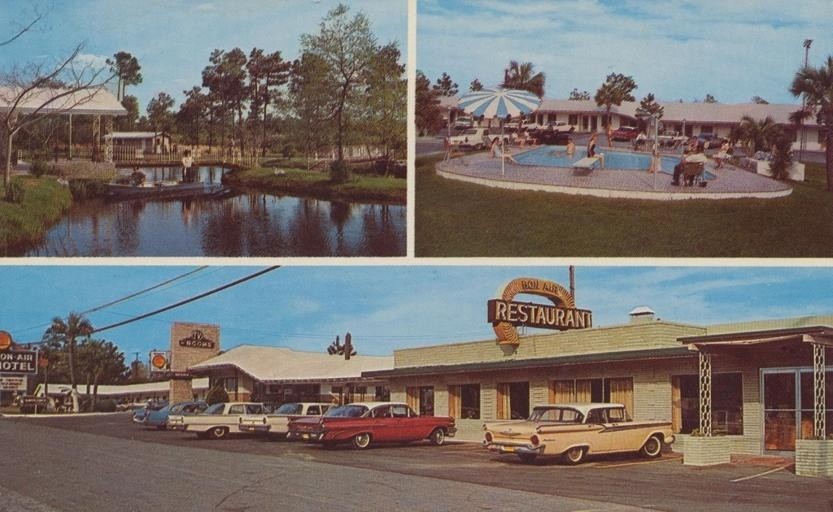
[505,119,538,130]
[614,126,728,149]
[132,402,456,449]
[456,117,473,127]
[540,121,576,132]
[480,403,676,465]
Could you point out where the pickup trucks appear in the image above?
[450,128,511,147]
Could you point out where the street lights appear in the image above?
[799,38,813,162]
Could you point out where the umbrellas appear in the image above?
[457,86,542,175]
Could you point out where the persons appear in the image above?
[566,138,575,160]
[481,127,537,166]
[180,199,193,226]
[131,165,146,186]
[586,133,605,170]
[180,149,193,183]
[633,127,734,187]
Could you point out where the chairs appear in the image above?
[445,139,466,166]
[682,160,703,186]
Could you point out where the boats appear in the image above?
[104,180,232,196]
